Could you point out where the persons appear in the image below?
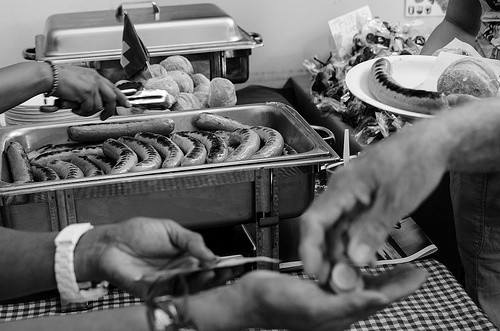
[419,0,500,60]
[299,94,500,331]
[0,60,131,121]
[0,217,428,331]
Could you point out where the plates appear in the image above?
[5,92,104,127]
[344,55,500,120]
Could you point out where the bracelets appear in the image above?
[43,60,59,105]
[148,290,198,331]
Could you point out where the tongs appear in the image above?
[38,79,176,112]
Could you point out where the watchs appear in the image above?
[53,222,111,304]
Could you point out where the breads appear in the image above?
[113,55,238,115]
[437,58,500,100]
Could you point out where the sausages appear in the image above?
[4,111,282,183]
[367,57,450,113]
